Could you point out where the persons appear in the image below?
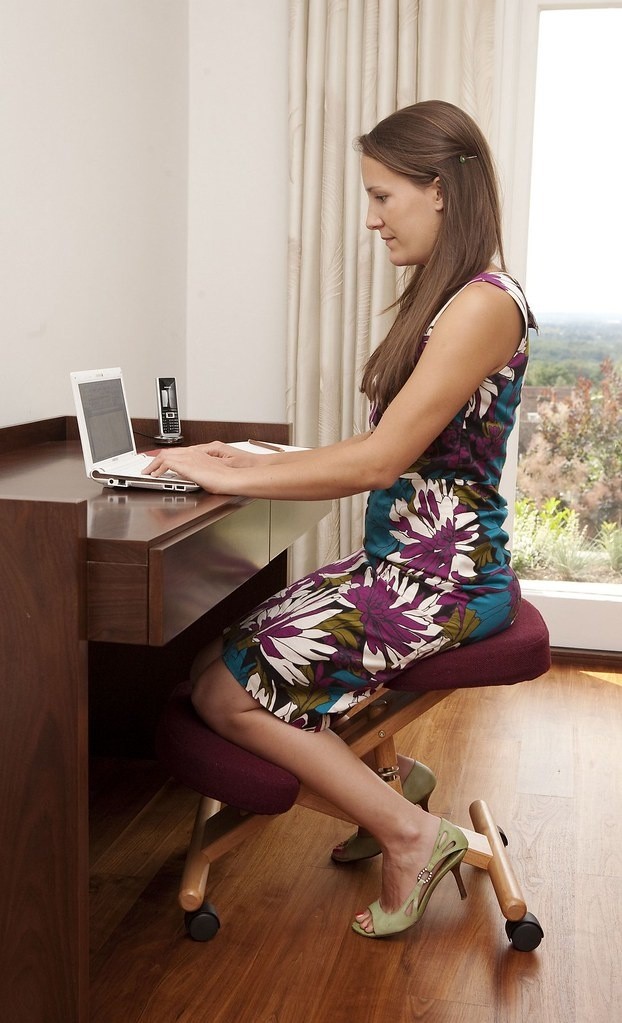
[141,100,537,936]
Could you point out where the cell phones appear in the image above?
[156,376,181,437]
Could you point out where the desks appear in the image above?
[0,417,332,1023]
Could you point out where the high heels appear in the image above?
[351,818,468,938]
[331,761,437,864]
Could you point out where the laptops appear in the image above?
[69,367,200,493]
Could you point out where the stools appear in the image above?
[149,560,551,952]
